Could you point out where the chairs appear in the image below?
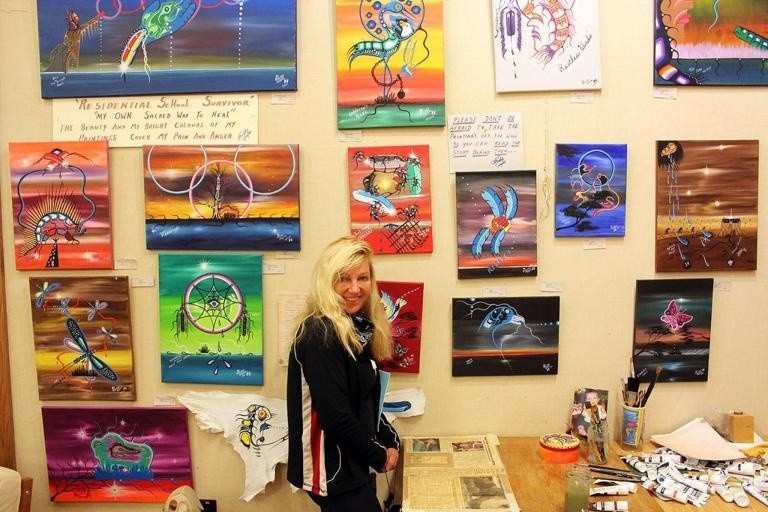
[0,466,33,511]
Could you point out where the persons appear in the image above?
[285,234,401,511]
[576,390,607,437]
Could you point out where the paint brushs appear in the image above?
[574,463,645,483]
[624,357,663,406]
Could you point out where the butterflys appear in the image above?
[660,299,693,331]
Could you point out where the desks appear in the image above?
[609,431,767,512]
[400,436,664,512]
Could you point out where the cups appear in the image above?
[587,425,607,465]
[564,466,590,512]
